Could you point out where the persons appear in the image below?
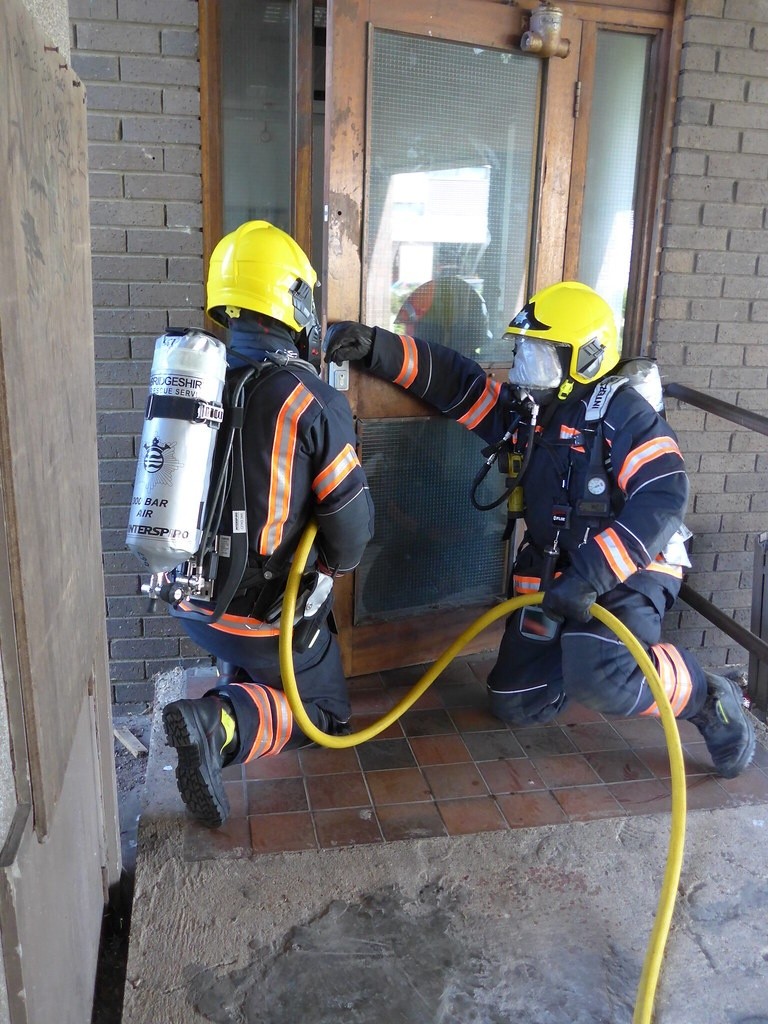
[393,276,487,361]
[323,283,755,779]
[127,219,375,826]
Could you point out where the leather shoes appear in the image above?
[687,671,756,779]
[216,658,253,685]
[162,693,231,829]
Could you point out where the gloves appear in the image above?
[321,321,375,367]
[542,565,598,624]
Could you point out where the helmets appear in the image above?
[393,277,494,339]
[206,220,318,333]
[501,281,621,385]
